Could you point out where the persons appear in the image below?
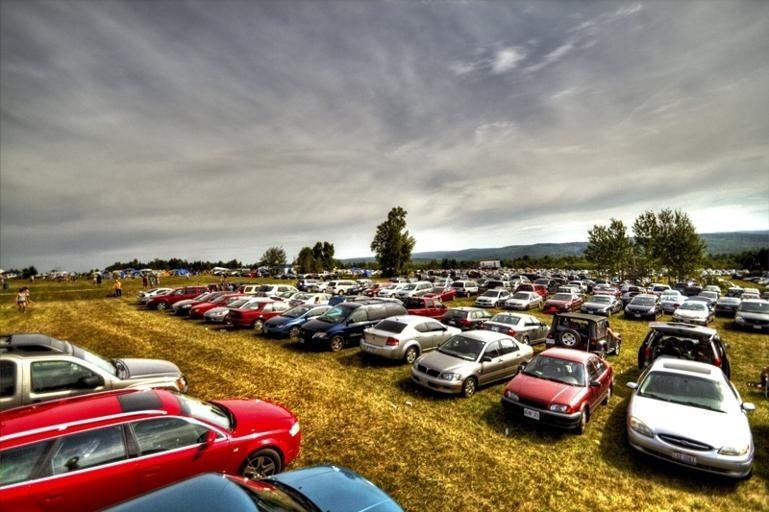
[113,279,122,296]
[16,289,26,313]
[24,287,34,304]
[143,273,160,289]
[97,274,101,286]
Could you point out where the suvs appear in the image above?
[543,312,623,360]
[636,321,731,382]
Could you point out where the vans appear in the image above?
[299,297,410,352]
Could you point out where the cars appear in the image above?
[661,296,688,313]
[734,298,769,334]
[543,293,584,312]
[503,344,611,434]
[482,309,553,347]
[1,392,302,511]
[715,296,740,316]
[138,264,369,340]
[369,264,769,302]
[410,329,536,399]
[96,459,406,512]
[360,315,464,359]
[1,330,189,408]
[624,293,665,320]
[671,301,711,326]
[621,354,757,482]
[578,295,624,316]
[504,291,544,309]
[473,289,510,307]
